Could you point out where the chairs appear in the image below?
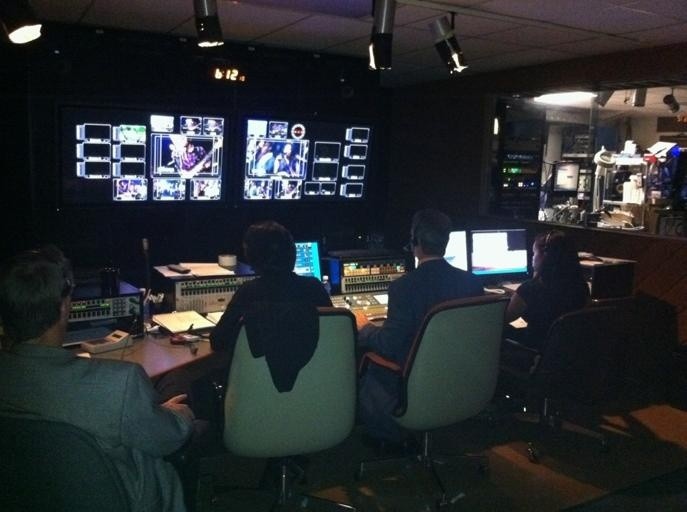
[352,289,513,511]
[0,413,208,512]
[186,303,363,511]
[497,300,650,464]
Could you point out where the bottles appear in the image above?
[321,276,330,297]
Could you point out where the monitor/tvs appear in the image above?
[552,161,581,197]
[414,227,468,272]
[234,102,380,212]
[469,227,532,285]
[291,240,323,282]
[55,100,233,213]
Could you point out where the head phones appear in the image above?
[543,231,558,266]
[19,248,72,297]
[412,210,443,246]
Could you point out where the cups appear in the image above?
[99,266,122,291]
[217,254,238,268]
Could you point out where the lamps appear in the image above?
[0,0,44,46]
[191,1,227,50]
[425,8,470,75]
[364,0,400,72]
[588,81,683,116]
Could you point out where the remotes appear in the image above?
[167,264,190,274]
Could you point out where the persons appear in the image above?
[273,142,301,178]
[251,141,274,176]
[209,218,336,490]
[504,227,593,367]
[356,206,485,486]
[0,239,195,512]
[182,142,202,171]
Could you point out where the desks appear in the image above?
[69,285,513,401]
